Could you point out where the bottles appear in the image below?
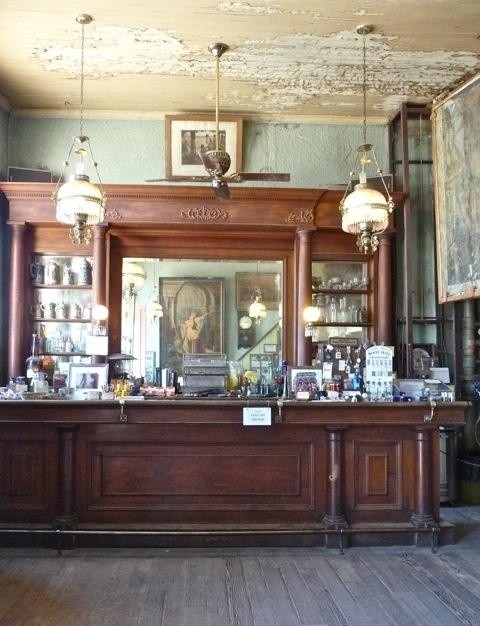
[77,257,92,286]
[315,296,369,324]
[29,262,43,286]
[62,268,74,286]
[44,259,60,285]
[24,334,43,379]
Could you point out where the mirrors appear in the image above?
[110,246,293,388]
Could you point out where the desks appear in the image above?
[1,397,472,549]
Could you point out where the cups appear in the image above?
[40,303,54,318]
[29,301,42,318]
[69,302,81,319]
[54,302,67,318]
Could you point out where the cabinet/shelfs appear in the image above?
[312,258,374,347]
[28,253,92,373]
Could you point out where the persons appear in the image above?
[182,310,210,353]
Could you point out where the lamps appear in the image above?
[90,304,109,336]
[51,14,106,245]
[249,260,266,325]
[121,262,163,323]
[109,354,137,379]
[339,24,394,255]
[304,306,318,337]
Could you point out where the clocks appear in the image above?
[237,311,255,349]
[400,342,435,379]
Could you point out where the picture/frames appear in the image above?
[68,364,108,389]
[164,115,244,178]
[158,277,227,368]
[430,67,480,305]
[236,272,280,311]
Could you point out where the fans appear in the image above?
[146,43,290,199]
